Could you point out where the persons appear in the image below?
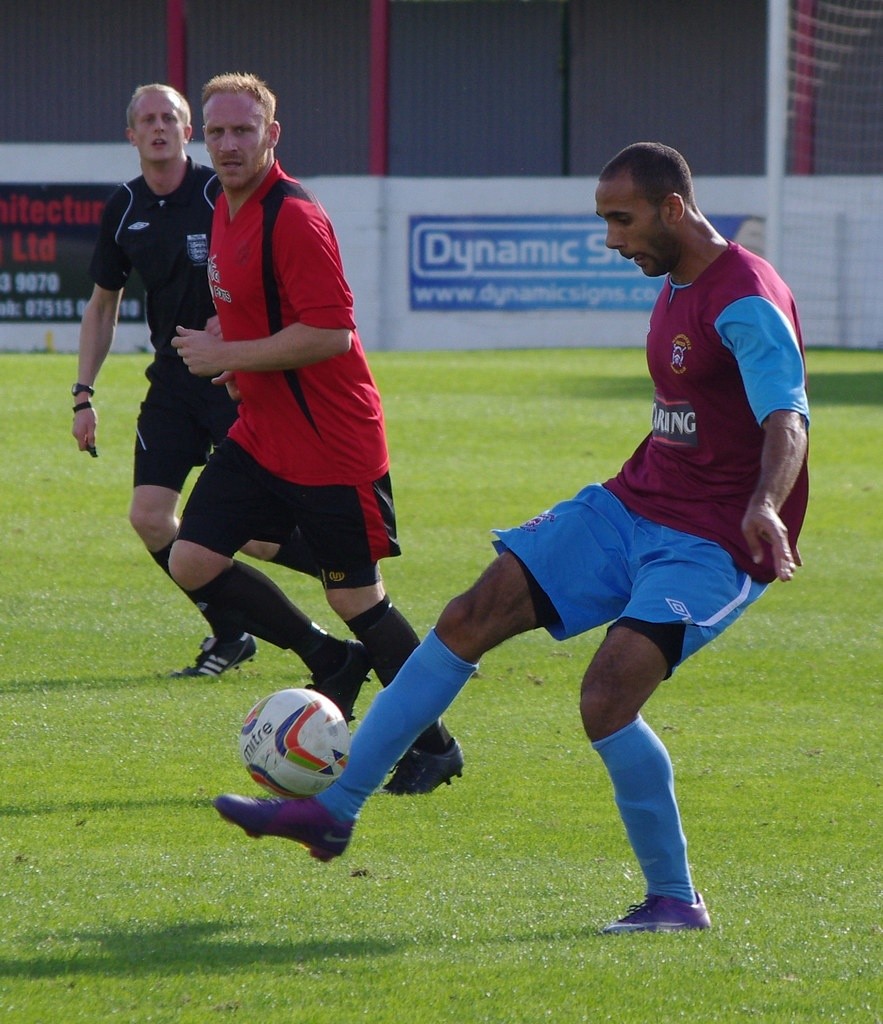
[170,75,465,798]
[71,81,324,678]
[206,137,812,937]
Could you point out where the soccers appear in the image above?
[240,687,351,798]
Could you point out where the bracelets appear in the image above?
[73,401,92,413]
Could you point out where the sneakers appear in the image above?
[214,792,355,862]
[173,634,259,678]
[382,739,465,796]
[309,640,375,724]
[603,891,713,934]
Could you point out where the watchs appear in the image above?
[71,383,96,398]
